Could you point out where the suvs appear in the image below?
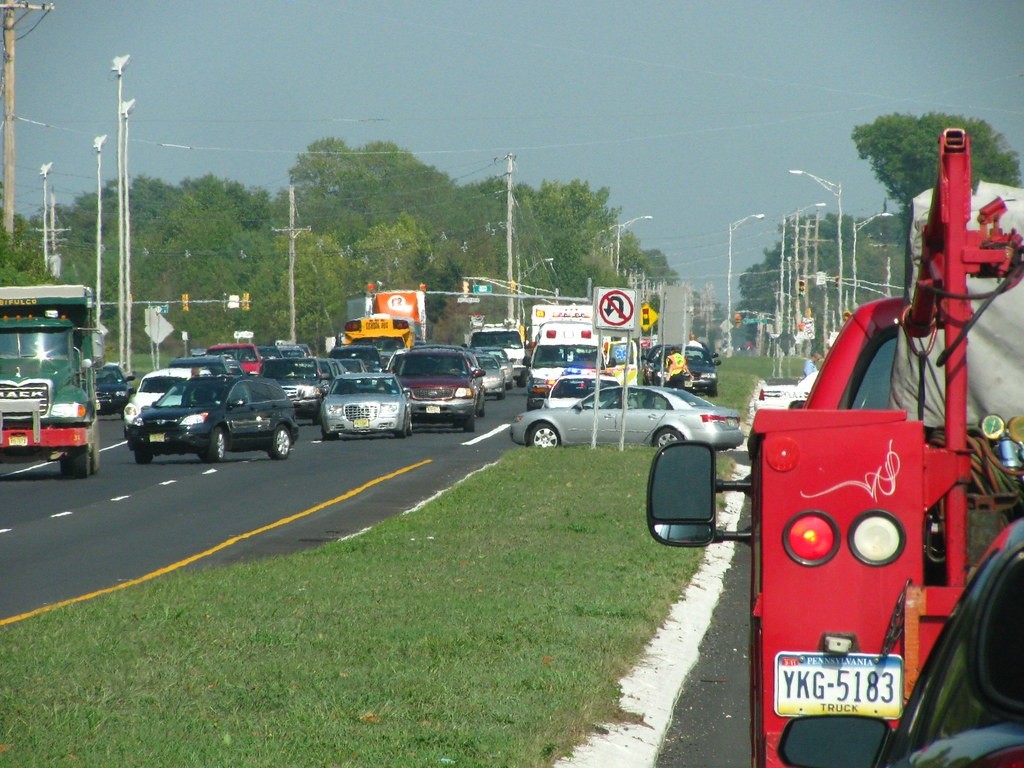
[169,342,312,375]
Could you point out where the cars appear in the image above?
[525,303,637,412]
[124,368,212,439]
[385,344,517,432]
[754,369,820,415]
[127,373,299,464]
[258,343,382,425]
[321,373,413,440]
[511,386,743,451]
[640,344,721,397]
[95,365,136,419]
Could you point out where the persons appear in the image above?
[688,334,701,348]
[802,352,821,378]
[665,347,695,389]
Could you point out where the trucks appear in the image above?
[0,285,98,478]
[465,315,526,387]
[344,291,426,370]
[645,129,1024,767]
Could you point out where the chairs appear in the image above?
[102,374,117,384]
[643,397,656,409]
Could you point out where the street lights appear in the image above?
[517,258,554,321]
[728,214,766,360]
[616,216,653,274]
[780,170,895,335]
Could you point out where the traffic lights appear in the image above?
[420,284,426,291]
[367,284,374,291]
